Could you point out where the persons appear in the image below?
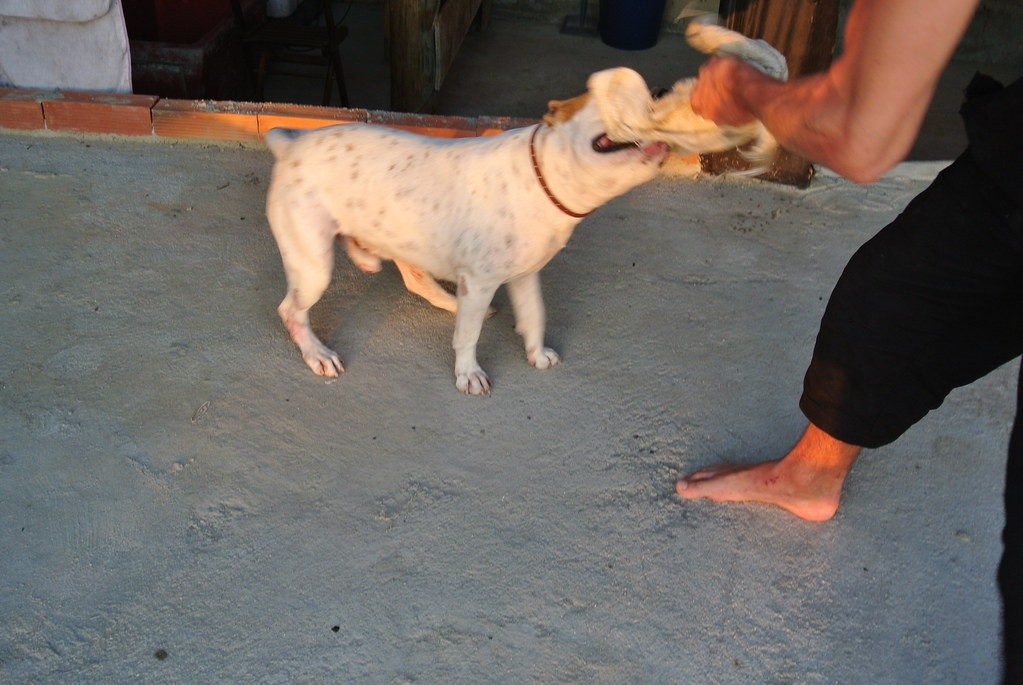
[675,0,1023,685]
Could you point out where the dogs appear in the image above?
[265,89,668,397]
